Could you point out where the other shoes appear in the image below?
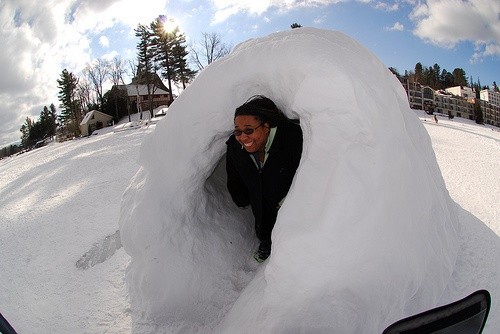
[253,250,272,263]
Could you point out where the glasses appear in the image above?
[232,123,264,137]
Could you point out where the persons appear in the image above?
[224,95,303,263]
